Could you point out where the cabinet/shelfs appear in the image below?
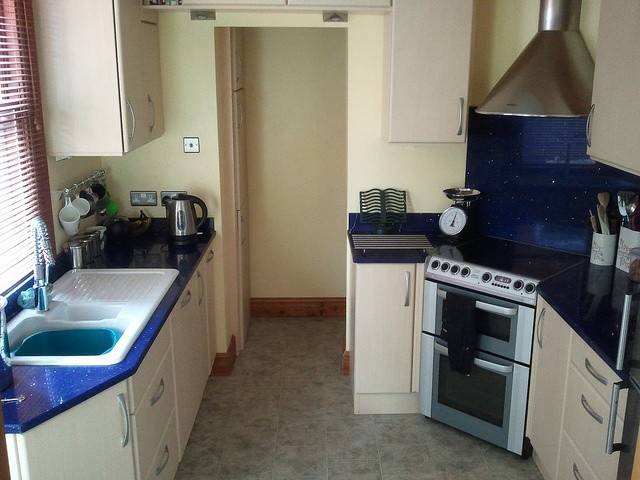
[555,331,627,480]
[353,262,416,395]
[220,88,248,211]
[584,0,640,176]
[226,197,250,354]
[34,0,166,157]
[172,244,208,460]
[220,27,244,93]
[126,319,180,480]
[381,0,474,144]
[17,381,139,479]
[203,244,218,375]
[525,294,573,480]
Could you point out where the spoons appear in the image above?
[624,194,640,229]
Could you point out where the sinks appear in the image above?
[1,314,136,359]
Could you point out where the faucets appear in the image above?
[31,217,55,312]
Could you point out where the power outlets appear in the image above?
[160,191,188,206]
[130,191,157,206]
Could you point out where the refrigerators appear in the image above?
[598,249,640,480]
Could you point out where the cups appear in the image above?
[71,192,91,219]
[83,186,100,206]
[58,197,81,235]
[90,182,110,202]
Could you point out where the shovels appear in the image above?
[618,190,637,229]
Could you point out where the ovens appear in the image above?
[417,289,536,458]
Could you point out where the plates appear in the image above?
[442,187,480,202]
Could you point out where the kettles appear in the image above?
[163,193,208,246]
